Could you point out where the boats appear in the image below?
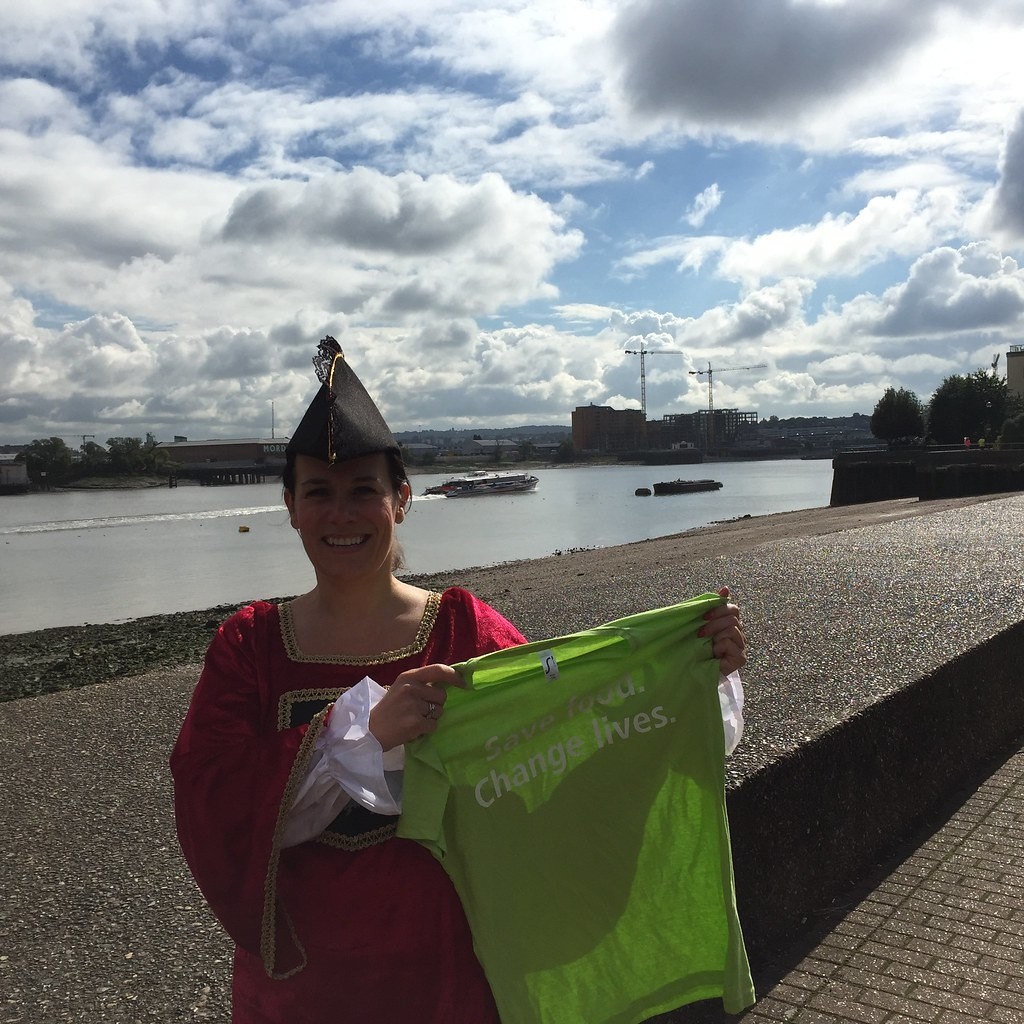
[653,477,723,495]
[424,469,539,498]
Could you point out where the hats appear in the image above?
[281,354,399,469]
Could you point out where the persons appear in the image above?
[964,437,971,453]
[168,334,749,1024]
[978,436,986,451]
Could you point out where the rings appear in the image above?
[425,702,436,720]
